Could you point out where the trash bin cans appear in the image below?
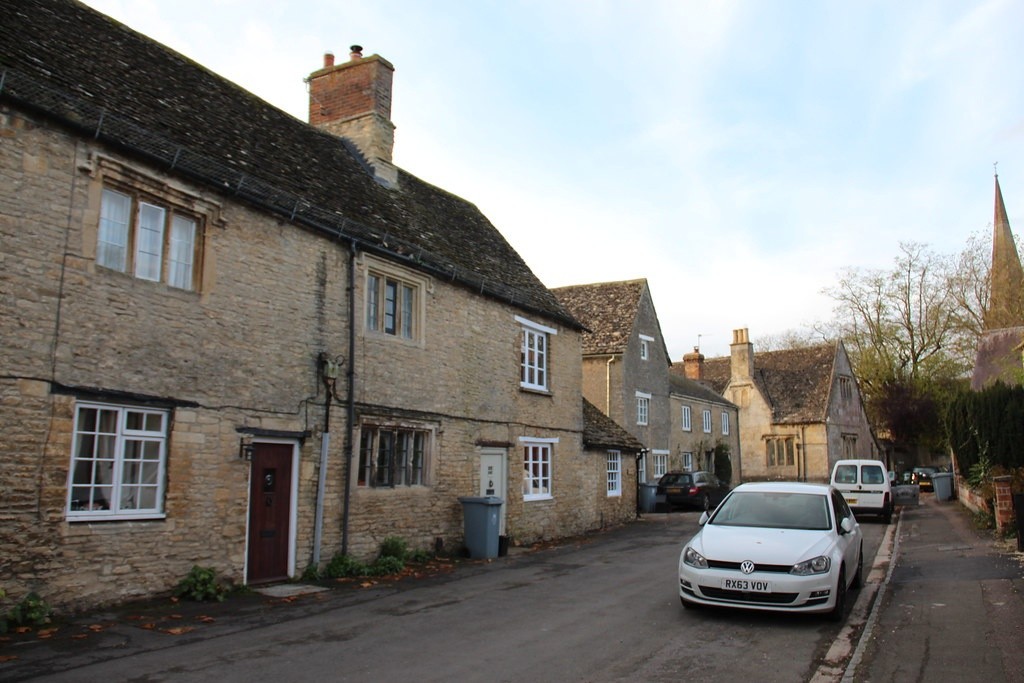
[457,496,505,561]
[931,473,953,502]
[638,483,659,513]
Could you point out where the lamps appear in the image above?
[238,437,255,460]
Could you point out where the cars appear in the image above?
[678,481,864,623]
[656,470,732,511]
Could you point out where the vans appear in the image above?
[827,458,896,525]
[887,467,937,493]
